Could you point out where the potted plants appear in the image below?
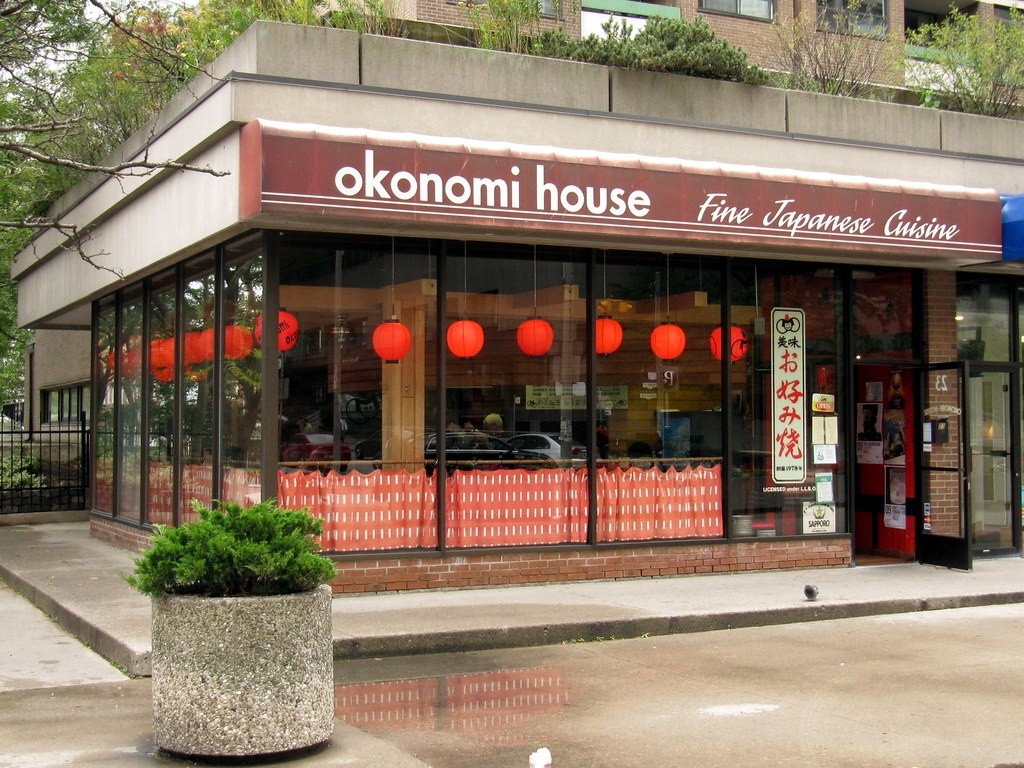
[117,495,338,765]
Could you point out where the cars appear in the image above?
[0,413,25,433]
[280,430,602,477]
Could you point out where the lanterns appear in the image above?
[595,315,624,358]
[709,323,748,366]
[107,307,299,382]
[516,316,555,359]
[372,319,411,364]
[649,321,687,362]
[446,317,486,361]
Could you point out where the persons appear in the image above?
[447,413,505,448]
[303,418,313,433]
[858,404,883,441]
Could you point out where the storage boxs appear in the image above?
[756,529,777,537]
[781,511,798,535]
